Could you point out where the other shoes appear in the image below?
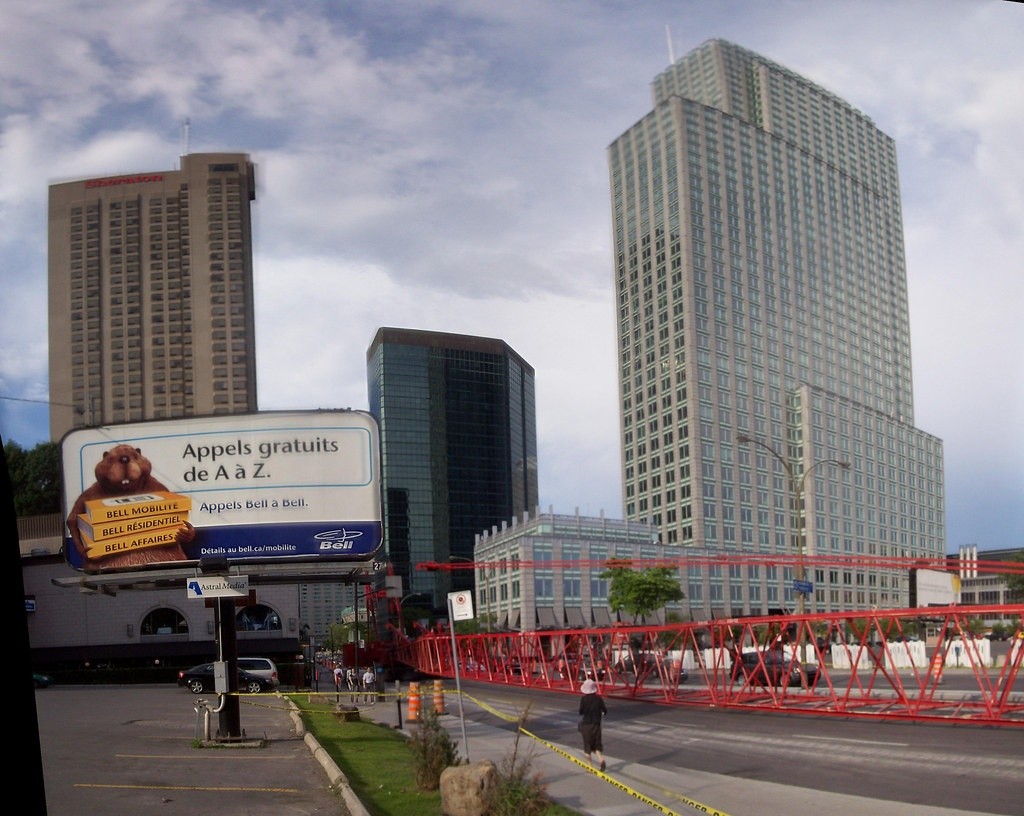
[601,761,606,771]
[371,702,374,704]
[364,701,366,704]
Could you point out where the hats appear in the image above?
[580,679,597,694]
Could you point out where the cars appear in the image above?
[177,661,274,694]
[559,659,606,682]
[33,673,53,688]
[615,652,689,684]
[236,656,280,688]
[728,650,821,686]
[458,659,486,674]
[495,656,524,676]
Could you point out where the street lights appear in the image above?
[735,435,852,690]
[353,584,396,677]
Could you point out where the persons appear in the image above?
[579,679,608,773]
[334,664,376,705]
